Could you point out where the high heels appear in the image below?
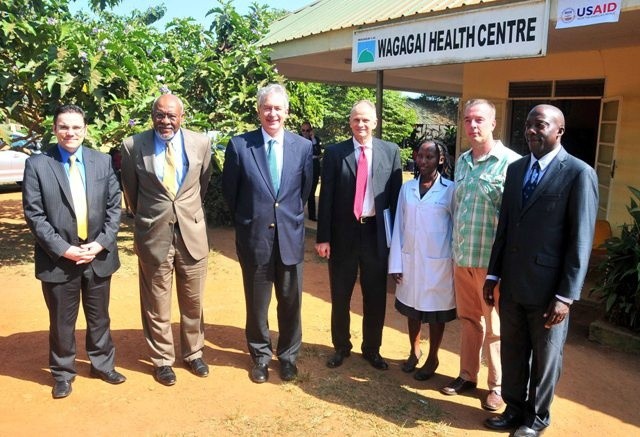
[415,360,439,381]
[402,350,423,371]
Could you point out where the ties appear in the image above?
[523,160,541,208]
[266,139,279,196]
[68,155,88,241]
[163,140,177,223]
[354,146,368,221]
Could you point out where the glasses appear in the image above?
[300,128,311,131]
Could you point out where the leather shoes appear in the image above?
[90,365,125,384]
[327,350,350,368]
[155,364,176,385]
[484,391,505,410]
[361,352,389,370]
[278,357,297,380]
[485,405,525,428]
[53,375,74,399]
[252,362,268,383]
[183,358,209,377]
[441,377,477,395]
[514,423,548,437]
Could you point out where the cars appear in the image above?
[0,132,43,186]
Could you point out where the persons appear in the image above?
[300,122,320,222]
[222,84,314,383]
[121,94,212,387]
[109,137,134,220]
[23,104,127,399]
[388,139,456,382]
[439,99,525,411]
[482,104,599,437]
[316,99,402,370]
